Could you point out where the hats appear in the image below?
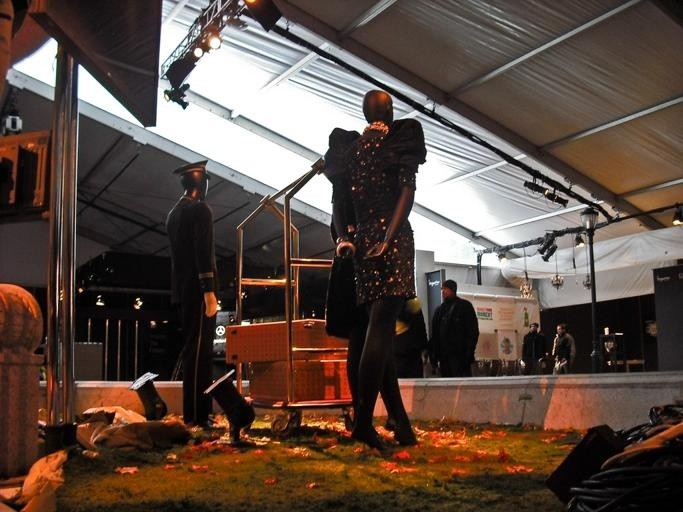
[442,279,459,295]
[172,158,212,176]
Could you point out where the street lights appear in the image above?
[578,207,604,372]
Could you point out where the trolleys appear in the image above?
[225,157,358,434]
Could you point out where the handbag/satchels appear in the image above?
[323,250,357,338]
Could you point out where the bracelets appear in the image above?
[384,238,394,246]
[336,241,356,257]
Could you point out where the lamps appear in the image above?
[549,238,564,292]
[158,0,285,112]
[580,233,593,292]
[518,246,535,299]
[518,166,574,209]
[667,201,683,227]
[532,231,586,263]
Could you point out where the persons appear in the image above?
[551,324,575,375]
[522,323,547,375]
[334,90,426,451]
[427,280,479,378]
[165,160,222,434]
[326,128,398,432]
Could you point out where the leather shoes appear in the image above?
[349,427,395,452]
[384,417,418,446]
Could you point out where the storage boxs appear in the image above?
[224,314,348,367]
[248,359,353,403]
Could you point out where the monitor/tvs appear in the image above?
[0,129,52,224]
[28,0,164,127]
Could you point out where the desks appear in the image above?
[604,356,646,373]
